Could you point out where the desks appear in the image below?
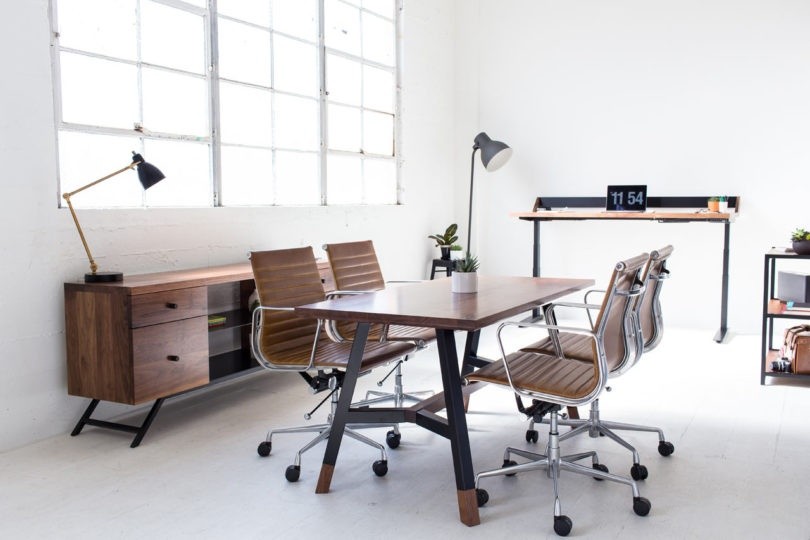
[294,274,596,528]
[514,196,740,343]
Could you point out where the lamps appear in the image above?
[466,132,513,252]
[62,149,166,282]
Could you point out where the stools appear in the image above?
[431,257,457,279]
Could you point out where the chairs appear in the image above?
[247,238,435,483]
[463,244,675,536]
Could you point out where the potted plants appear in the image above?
[427,224,462,260]
[791,227,810,255]
[452,254,480,294]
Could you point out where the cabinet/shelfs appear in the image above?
[761,246,810,385]
[64,261,335,448]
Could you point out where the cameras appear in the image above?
[770,358,791,373]
